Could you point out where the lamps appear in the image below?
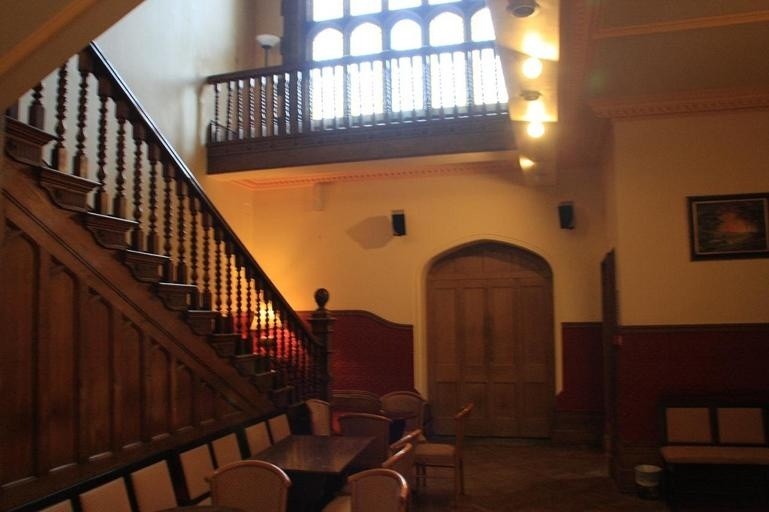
[250,303,282,347]
[256,33,280,137]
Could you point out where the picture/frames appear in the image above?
[686,191,769,259]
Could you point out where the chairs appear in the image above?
[203,391,475,512]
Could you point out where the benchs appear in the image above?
[656,398,769,512]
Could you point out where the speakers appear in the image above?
[558,201,575,230]
[392,213,405,236]
